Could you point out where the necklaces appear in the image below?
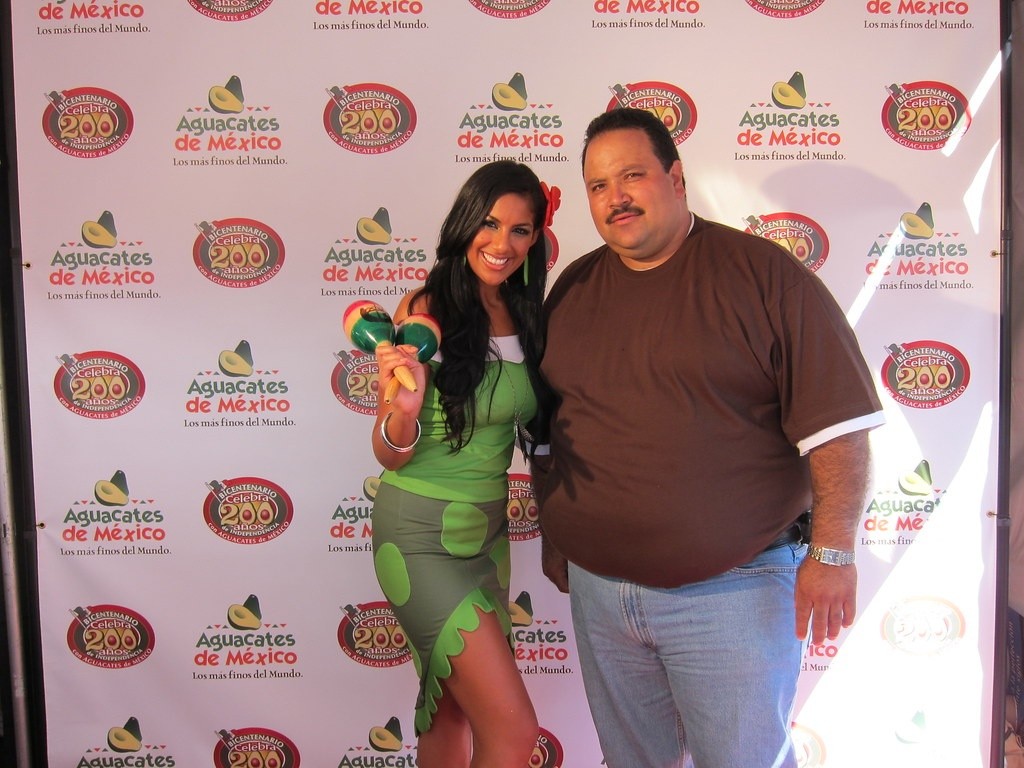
[483,340,534,441]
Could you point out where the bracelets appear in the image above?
[380,412,422,453]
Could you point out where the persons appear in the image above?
[530,109,889,768]
[370,159,545,768]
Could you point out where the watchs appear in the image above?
[807,541,855,567]
